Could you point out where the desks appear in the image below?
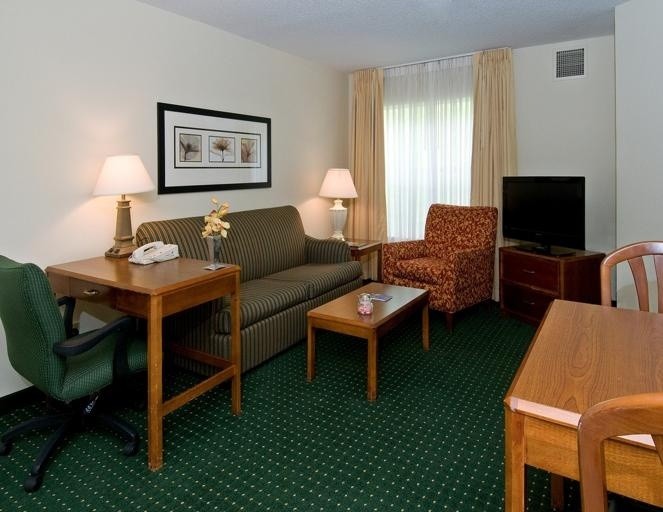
[44,253,242,470]
[345,239,384,284]
[503,298,663,512]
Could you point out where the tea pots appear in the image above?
[355,293,374,315]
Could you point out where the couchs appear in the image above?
[134,206,364,378]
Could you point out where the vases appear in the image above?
[202,233,230,270]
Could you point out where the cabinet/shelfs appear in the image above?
[499,244,607,327]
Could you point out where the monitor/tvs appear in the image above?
[502,176,585,257]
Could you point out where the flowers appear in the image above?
[202,199,233,238]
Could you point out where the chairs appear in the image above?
[577,393,663,512]
[600,240,663,313]
[0,255,178,493]
[383,203,498,330]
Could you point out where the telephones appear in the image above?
[128,241,179,264]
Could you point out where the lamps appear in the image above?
[320,167,358,241]
[92,154,155,258]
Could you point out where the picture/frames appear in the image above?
[158,100,272,197]
